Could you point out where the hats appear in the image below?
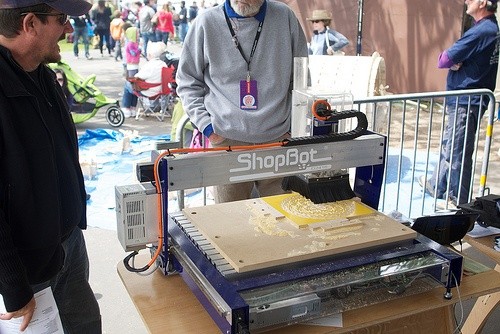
[0,0,93,16]
[306,9,333,20]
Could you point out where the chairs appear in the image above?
[129,65,174,121]
[392,205,481,252]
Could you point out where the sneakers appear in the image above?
[433,201,457,211]
[121,108,136,117]
[417,174,442,199]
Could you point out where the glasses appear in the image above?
[312,20,320,23]
[20,12,69,26]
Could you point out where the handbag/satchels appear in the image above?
[333,49,346,56]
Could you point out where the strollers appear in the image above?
[49,61,126,128]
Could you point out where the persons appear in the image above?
[68,0,226,119]
[52,68,73,111]
[175,0,311,206]
[0,0,103,334]
[418,0,500,211]
[306,10,350,56]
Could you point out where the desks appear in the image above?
[117,252,456,334]
